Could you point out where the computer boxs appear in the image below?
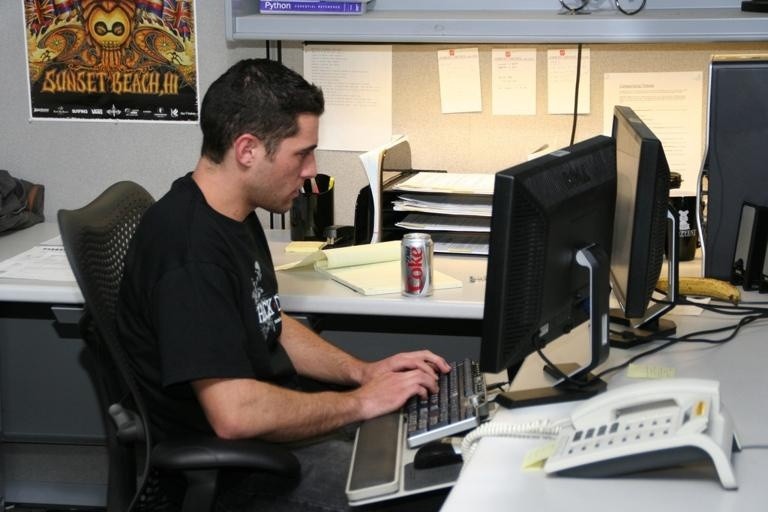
[696,62,768,281]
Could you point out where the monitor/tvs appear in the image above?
[479,134,617,410]
[607,106,680,350]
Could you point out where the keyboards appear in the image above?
[407,357,489,449]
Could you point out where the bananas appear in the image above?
[655,277,741,306]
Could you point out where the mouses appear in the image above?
[414,437,465,469]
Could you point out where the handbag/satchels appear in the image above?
[0,168,46,239]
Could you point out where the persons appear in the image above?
[115,58,450,511]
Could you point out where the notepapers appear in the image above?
[285,240,325,254]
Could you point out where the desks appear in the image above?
[438,247,768,512]
[1,221,488,506]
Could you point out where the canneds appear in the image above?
[401,232,435,297]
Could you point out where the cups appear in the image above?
[289,175,335,242]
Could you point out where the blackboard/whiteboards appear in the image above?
[224,3,618,43]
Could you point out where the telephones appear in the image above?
[546,378,742,490]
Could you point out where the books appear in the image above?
[358,133,409,244]
[275,239,463,295]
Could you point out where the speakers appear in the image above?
[729,201,768,291]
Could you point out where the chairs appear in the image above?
[57,181,302,512]
